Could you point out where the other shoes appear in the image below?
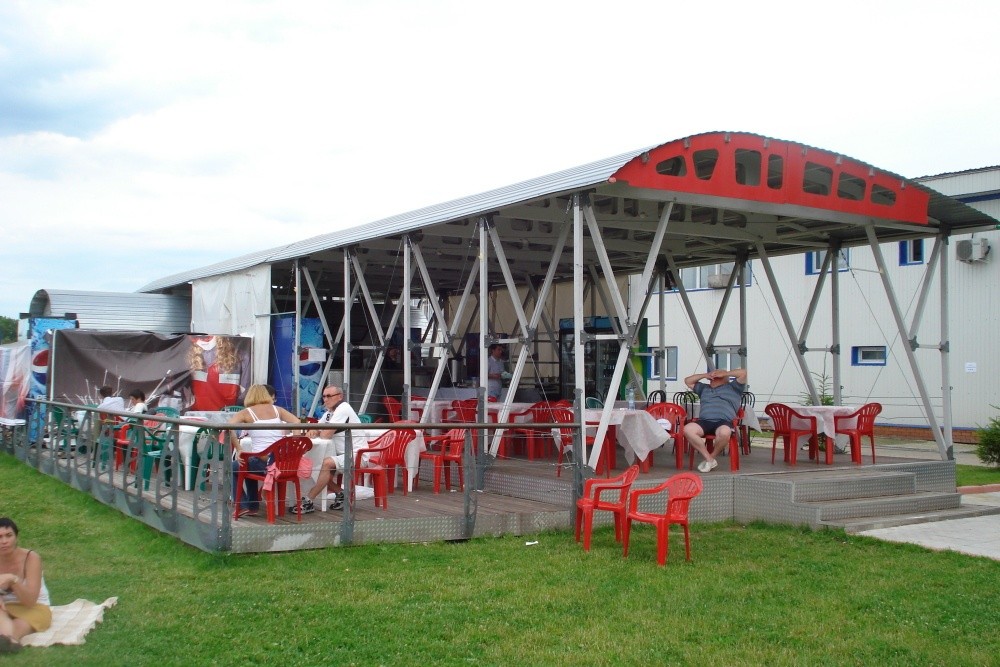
[232,506,250,517]
[244,508,258,515]
[0,634,26,654]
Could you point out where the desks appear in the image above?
[474,403,536,430]
[364,429,427,491]
[166,426,225,491]
[410,400,457,450]
[680,403,762,455]
[231,437,337,515]
[767,405,862,465]
[551,408,671,470]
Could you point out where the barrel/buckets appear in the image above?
[471,377,479,388]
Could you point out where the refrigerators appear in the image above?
[271,316,323,421]
[559,316,647,440]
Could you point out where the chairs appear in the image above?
[52,390,882,566]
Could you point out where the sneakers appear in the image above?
[697,459,706,471]
[288,497,315,514]
[330,490,344,509]
[700,459,718,472]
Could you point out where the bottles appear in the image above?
[627,333,644,401]
[300,408,308,435]
[628,389,636,411]
[603,364,615,378]
[602,343,620,361]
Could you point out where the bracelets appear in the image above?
[11,575,18,586]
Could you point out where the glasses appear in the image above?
[323,392,340,398]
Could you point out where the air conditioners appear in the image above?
[954,238,988,262]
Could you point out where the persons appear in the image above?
[487,344,507,402]
[227,382,302,516]
[91,386,125,427]
[127,389,148,423]
[0,517,51,654]
[289,384,369,514]
[681,368,746,473]
